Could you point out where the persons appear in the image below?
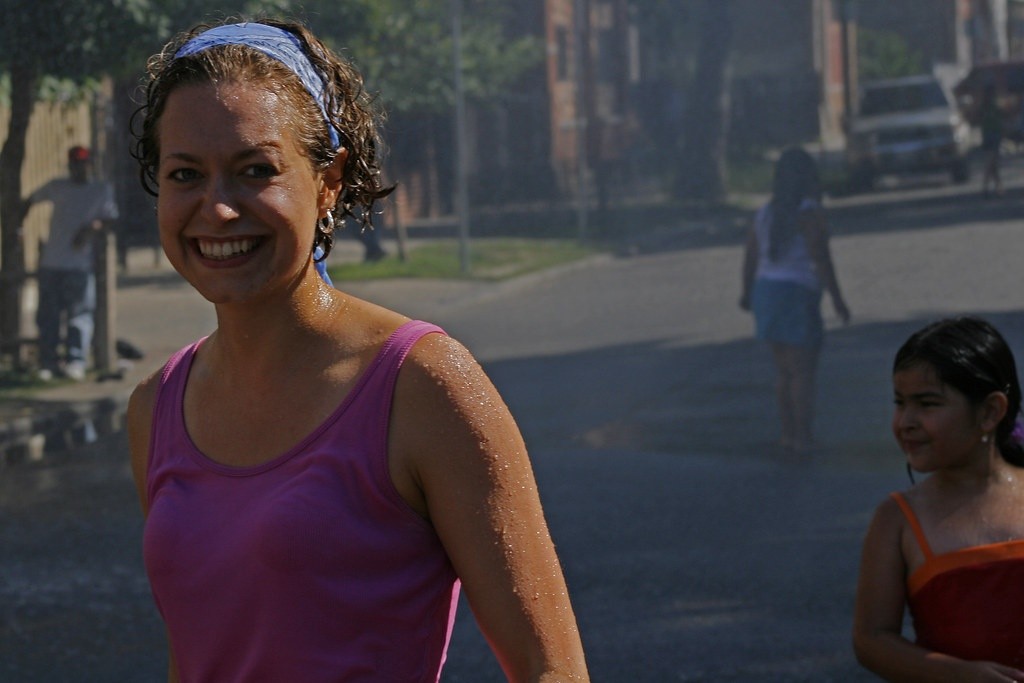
[853,317,1024,683]
[125,19,591,683]
[12,146,123,379]
[740,148,850,451]
[965,94,1010,201]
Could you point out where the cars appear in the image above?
[846,74,972,192]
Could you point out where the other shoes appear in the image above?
[794,438,827,453]
[782,436,794,448]
[36,368,52,381]
[64,363,85,379]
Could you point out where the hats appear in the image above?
[68,146,89,160]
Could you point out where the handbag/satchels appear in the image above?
[739,242,758,309]
